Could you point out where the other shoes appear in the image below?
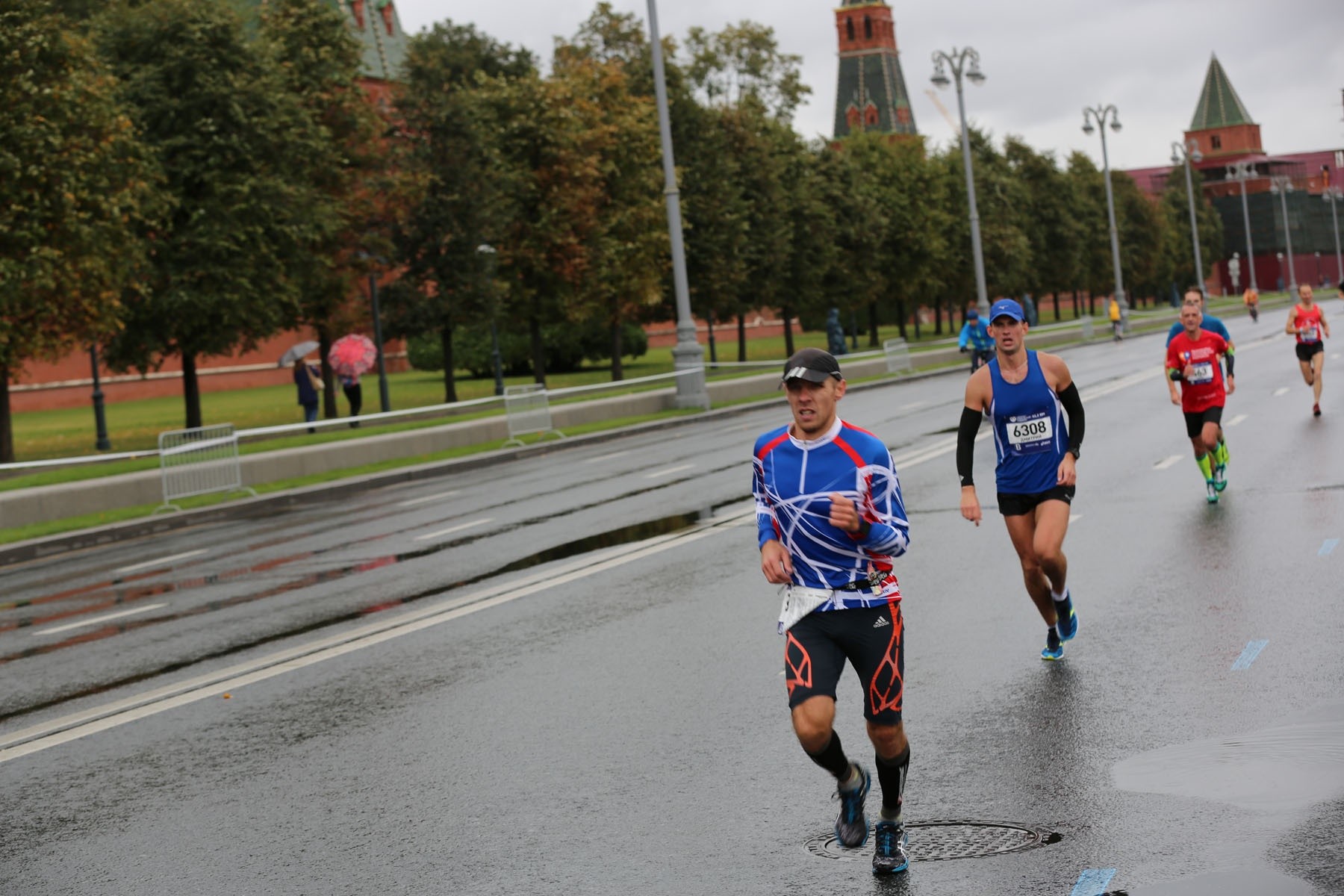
[308,426,316,434]
[1313,403,1321,417]
[1309,368,1314,386]
[1221,439,1230,463]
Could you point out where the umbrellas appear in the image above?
[279,340,321,368]
[329,334,378,377]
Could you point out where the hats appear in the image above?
[967,310,978,320]
[778,347,842,391]
[989,298,1026,326]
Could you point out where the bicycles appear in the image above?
[963,347,993,374]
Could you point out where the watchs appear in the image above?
[1068,447,1080,460]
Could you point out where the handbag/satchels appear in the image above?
[306,366,325,391]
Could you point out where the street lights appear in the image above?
[1082,104,1129,318]
[931,45,991,320]
[1225,158,1260,294]
[1322,184,1344,283]
[1171,138,1209,304]
[1268,173,1300,302]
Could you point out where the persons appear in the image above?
[293,358,320,434]
[1168,282,1343,417]
[1109,294,1124,342]
[335,376,364,429]
[956,300,1086,658]
[1167,303,1235,505]
[959,310,995,371]
[750,347,911,872]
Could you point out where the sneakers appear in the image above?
[1051,586,1079,641]
[1206,480,1219,504]
[871,820,908,874]
[1213,460,1227,492]
[831,759,872,849]
[1041,626,1064,660]
